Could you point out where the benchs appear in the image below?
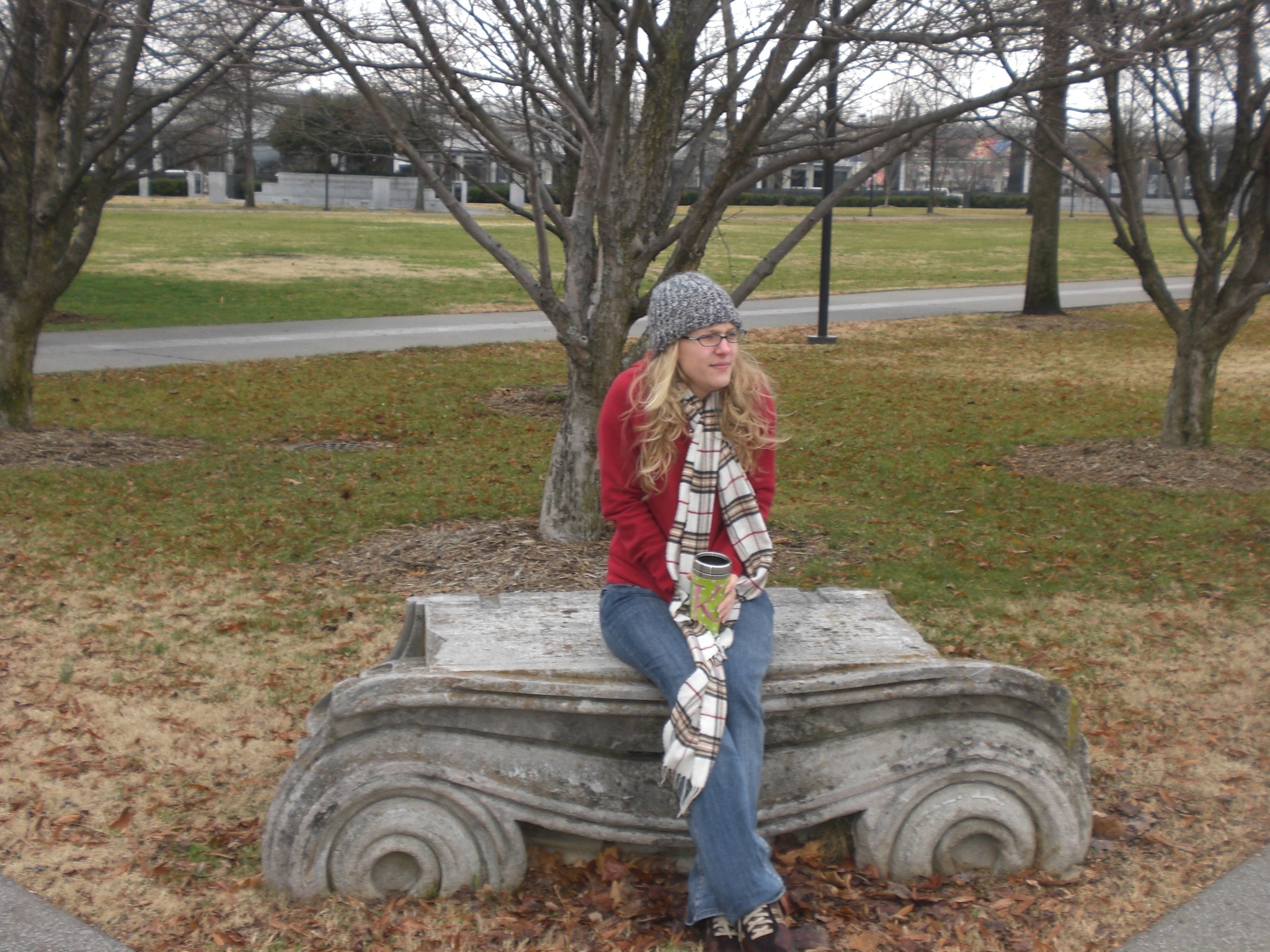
[259,589,1096,907]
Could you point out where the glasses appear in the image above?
[682,330,746,347]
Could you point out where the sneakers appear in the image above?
[742,902,792,952]
[705,914,744,952]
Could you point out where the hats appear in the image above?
[648,272,742,353]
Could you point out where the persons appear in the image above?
[600,268,794,952]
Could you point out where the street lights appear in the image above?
[858,109,873,216]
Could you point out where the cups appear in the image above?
[690,552,732,636]
